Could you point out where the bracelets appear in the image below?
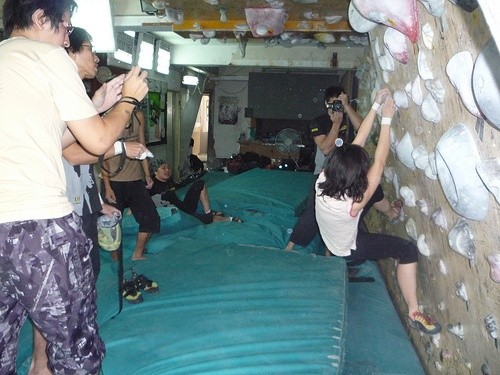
[118,96,140,105]
[371,102,380,111]
[114,141,126,154]
[381,117,392,125]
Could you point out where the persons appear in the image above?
[315,86,442,335]
[285,85,403,250]
[146,157,233,224]
[28,26,154,375]
[99,109,160,262]
[0,0,150,375]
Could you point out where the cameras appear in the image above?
[326,100,344,112]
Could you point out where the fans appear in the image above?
[275,127,302,170]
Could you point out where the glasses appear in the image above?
[79,43,96,56]
[43,10,73,36]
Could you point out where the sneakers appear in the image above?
[409,312,441,335]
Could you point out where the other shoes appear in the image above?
[134,275,158,291]
[230,217,241,223]
[206,211,224,216]
[121,281,142,301]
